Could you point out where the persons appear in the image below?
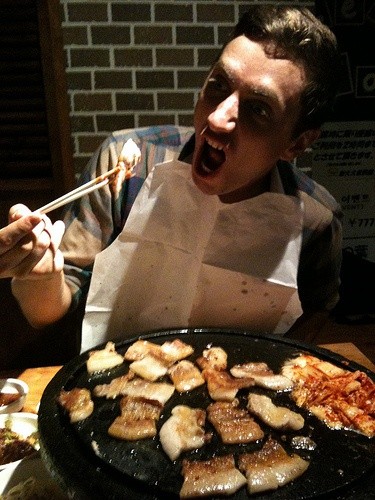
[0,4,375,375]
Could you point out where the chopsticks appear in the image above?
[34,167,116,213]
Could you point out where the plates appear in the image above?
[0,412,38,470]
[38,327,375,500]
[0,378,28,414]
